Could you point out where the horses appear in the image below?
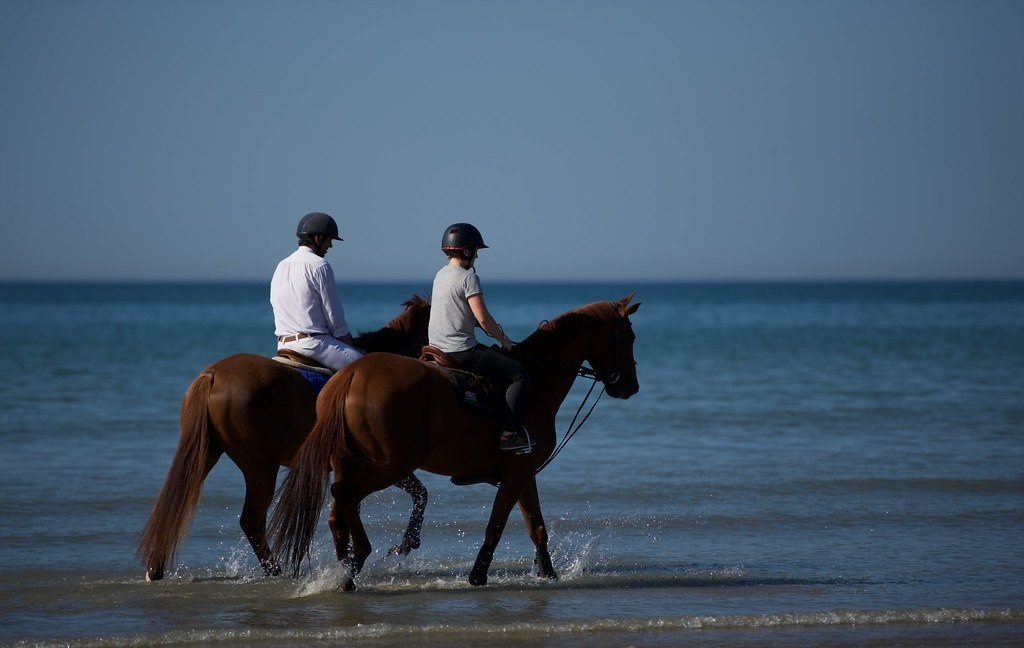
[129,292,431,581]
[259,292,641,590]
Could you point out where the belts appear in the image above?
[278,333,327,342]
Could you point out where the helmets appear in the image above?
[441,223,489,251]
[297,212,344,241]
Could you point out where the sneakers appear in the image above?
[500,432,536,451]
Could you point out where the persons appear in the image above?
[269,212,366,371]
[428,222,538,451]
